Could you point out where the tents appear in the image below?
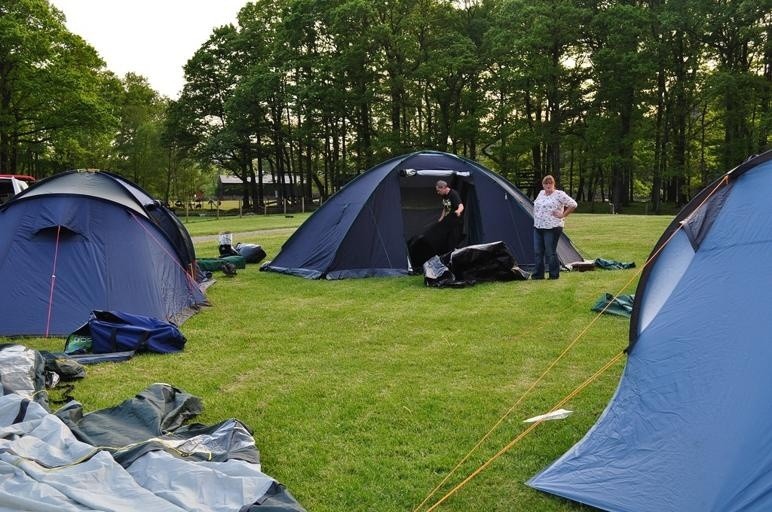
[521,149,772,510]
[259,148,597,281]
[1,167,218,344]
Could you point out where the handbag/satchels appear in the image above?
[89,308,188,353]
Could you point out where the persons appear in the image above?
[435,178,465,239]
[530,174,579,281]
